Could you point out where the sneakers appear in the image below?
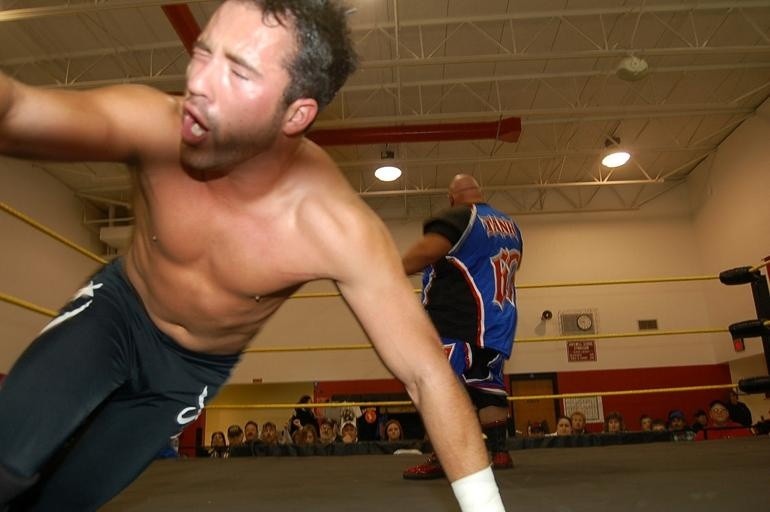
[402,453,442,480]
[492,451,513,468]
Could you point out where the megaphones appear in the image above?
[541,310,553,321]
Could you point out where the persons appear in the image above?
[210,420,284,459]
[0,0,506,512]
[285,396,403,459]
[602,411,626,433]
[641,396,753,442]
[527,412,588,434]
[401,174,522,480]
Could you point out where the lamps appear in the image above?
[615,55,649,82]
[374,143,402,182]
[601,136,631,168]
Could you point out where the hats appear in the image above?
[227,424,242,437]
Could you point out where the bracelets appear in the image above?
[450,468,505,512]
